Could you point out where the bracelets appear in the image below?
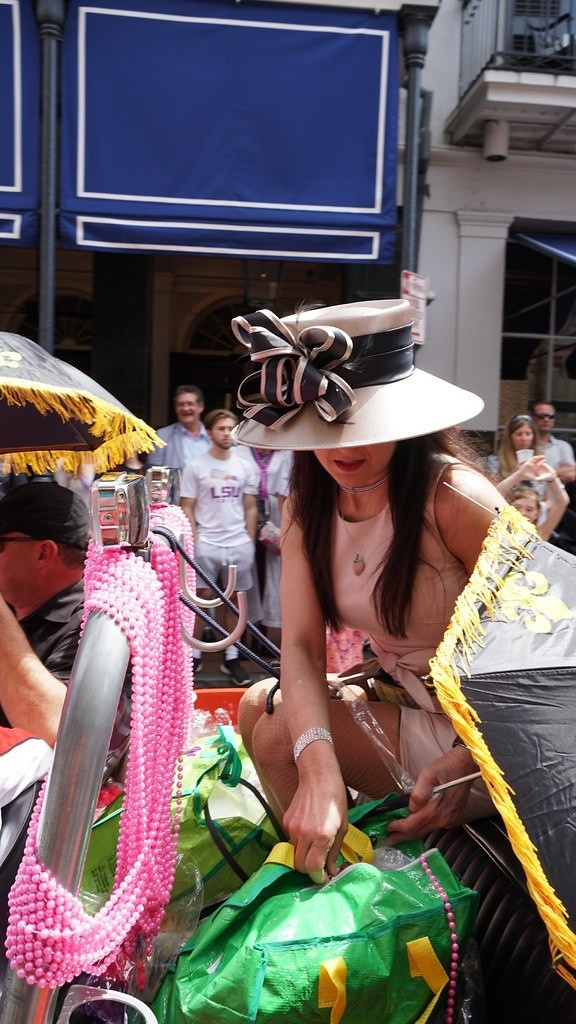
[294,727,334,765]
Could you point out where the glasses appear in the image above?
[512,416,533,423]
[532,413,556,419]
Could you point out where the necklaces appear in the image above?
[3,503,195,988]
[334,476,389,576]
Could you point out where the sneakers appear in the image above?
[221,657,251,686]
[191,658,202,683]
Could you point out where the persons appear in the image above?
[144,386,215,502]
[179,410,293,686]
[531,394,576,481]
[497,414,548,525]
[0,484,96,751]
[493,458,570,540]
[237,309,512,876]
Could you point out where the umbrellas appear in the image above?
[423,504,576,988]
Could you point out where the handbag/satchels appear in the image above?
[144,789,478,1024]
[80,724,280,917]
[257,521,282,557]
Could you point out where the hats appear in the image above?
[0,481,91,549]
[229,298,485,451]
